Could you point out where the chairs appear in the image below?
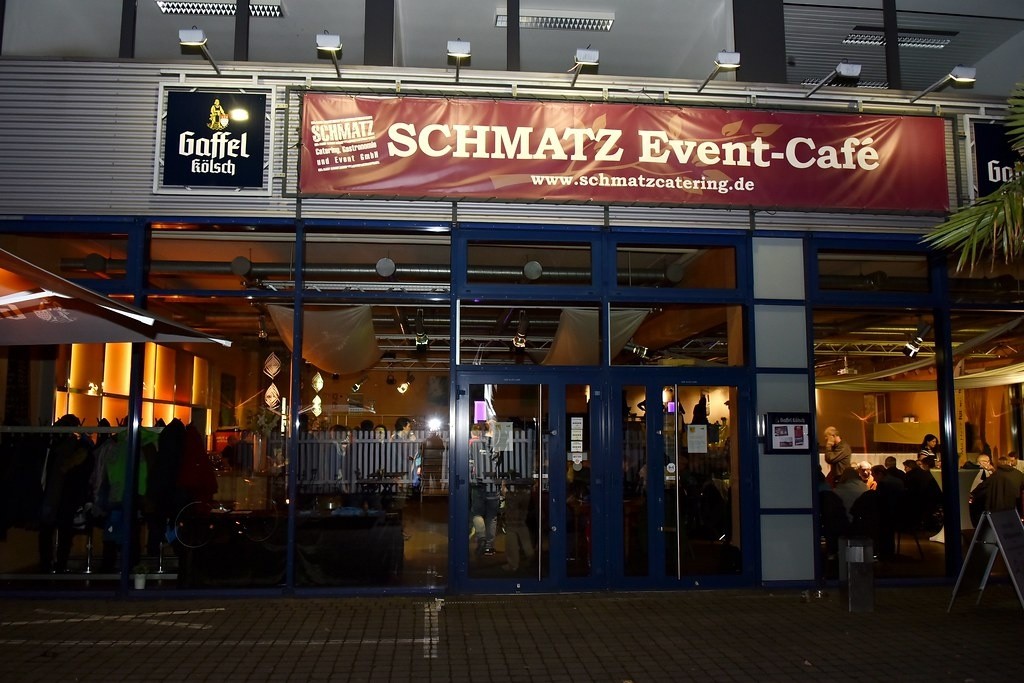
[296,469,376,502]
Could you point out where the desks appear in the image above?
[193,509,401,584]
[368,471,407,497]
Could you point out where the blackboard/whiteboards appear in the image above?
[946,509,1024,610]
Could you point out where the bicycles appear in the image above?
[172,477,280,549]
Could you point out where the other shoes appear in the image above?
[485,547,495,555]
[500,563,518,573]
[475,536,486,555]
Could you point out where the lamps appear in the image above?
[315,29,343,78]
[522,259,544,279]
[86,252,106,273]
[446,38,471,82]
[571,44,600,87]
[178,25,220,76]
[375,258,395,277]
[257,318,268,338]
[806,59,862,97]
[299,351,415,394]
[230,256,252,277]
[859,270,888,287]
[836,357,857,376]
[512,317,529,348]
[910,64,977,103]
[414,314,428,346]
[904,323,932,358]
[697,49,741,92]
[623,337,648,358]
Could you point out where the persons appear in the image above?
[818,426,944,575]
[469,420,500,555]
[623,448,704,562]
[917,434,1024,530]
[502,476,534,571]
[299,412,412,482]
[221,430,254,471]
